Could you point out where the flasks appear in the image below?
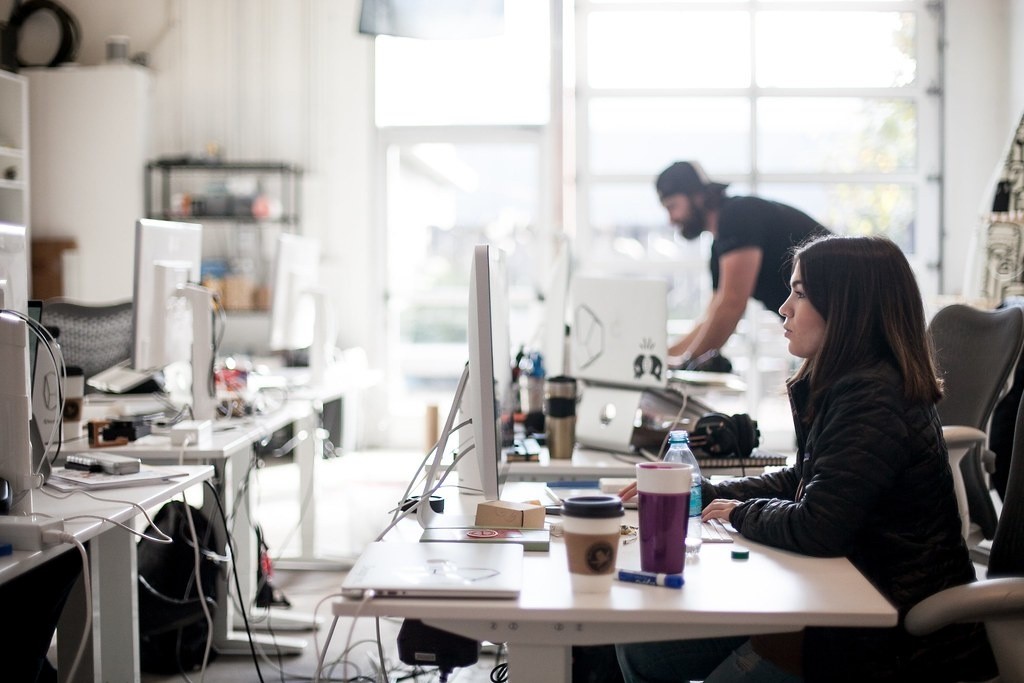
[545,382,576,460]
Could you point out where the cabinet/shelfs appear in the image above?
[0,70,33,322]
[144,162,310,463]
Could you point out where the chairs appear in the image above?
[906,394,1024,683]
[928,302,1024,541]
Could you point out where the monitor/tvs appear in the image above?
[0,222,32,513]
[132,214,219,429]
[417,245,546,531]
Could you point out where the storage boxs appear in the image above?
[475,499,546,529]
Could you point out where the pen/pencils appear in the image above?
[626,537,638,544]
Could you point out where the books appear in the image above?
[47,464,189,492]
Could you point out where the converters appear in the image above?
[0,515,67,552]
[170,419,213,446]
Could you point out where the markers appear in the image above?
[618,569,686,590]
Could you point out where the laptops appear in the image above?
[340,540,523,602]
[570,278,718,417]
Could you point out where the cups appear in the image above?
[636,461,694,575]
[61,366,85,440]
[560,493,626,595]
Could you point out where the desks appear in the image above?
[330,391,898,683]
[0,375,348,683]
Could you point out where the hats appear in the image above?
[656,160,728,199]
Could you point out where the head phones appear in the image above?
[685,412,761,459]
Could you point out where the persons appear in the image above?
[655,160,833,369]
[571,236,1001,683]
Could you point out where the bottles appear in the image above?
[663,429,703,556]
[250,175,283,221]
[32,324,62,443]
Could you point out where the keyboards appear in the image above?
[700,517,735,545]
